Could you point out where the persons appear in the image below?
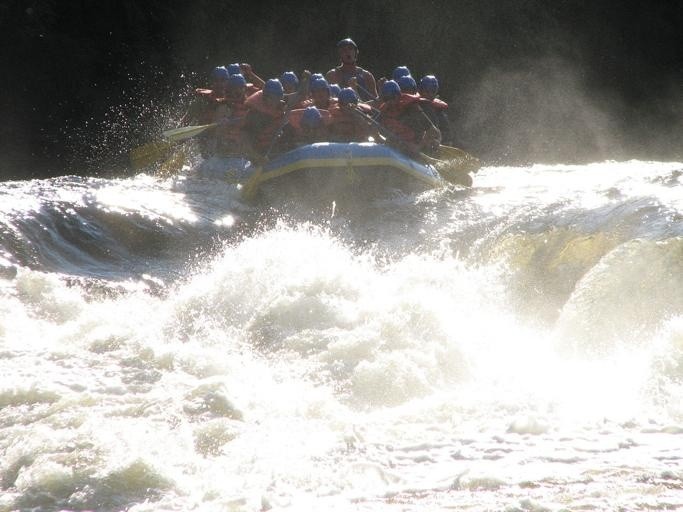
[325,38,376,102]
[189,62,451,165]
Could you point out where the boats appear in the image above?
[234,141,454,209]
[189,152,257,190]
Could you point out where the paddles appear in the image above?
[353,108,472,187]
[239,70,312,202]
[433,144,480,175]
[162,123,220,140]
[130,140,182,169]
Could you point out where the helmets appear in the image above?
[214,36,442,129]
